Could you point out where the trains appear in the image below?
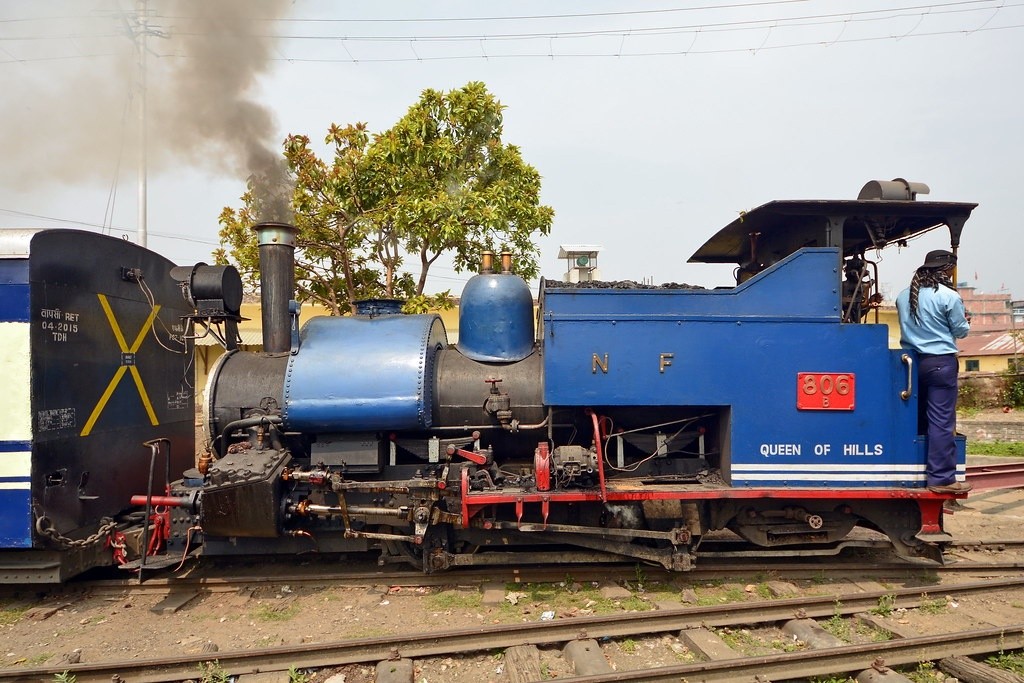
[0,179,980,581]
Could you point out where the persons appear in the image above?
[895,250,972,493]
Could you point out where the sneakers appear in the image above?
[928,482,973,492]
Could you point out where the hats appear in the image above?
[922,249,957,269]
